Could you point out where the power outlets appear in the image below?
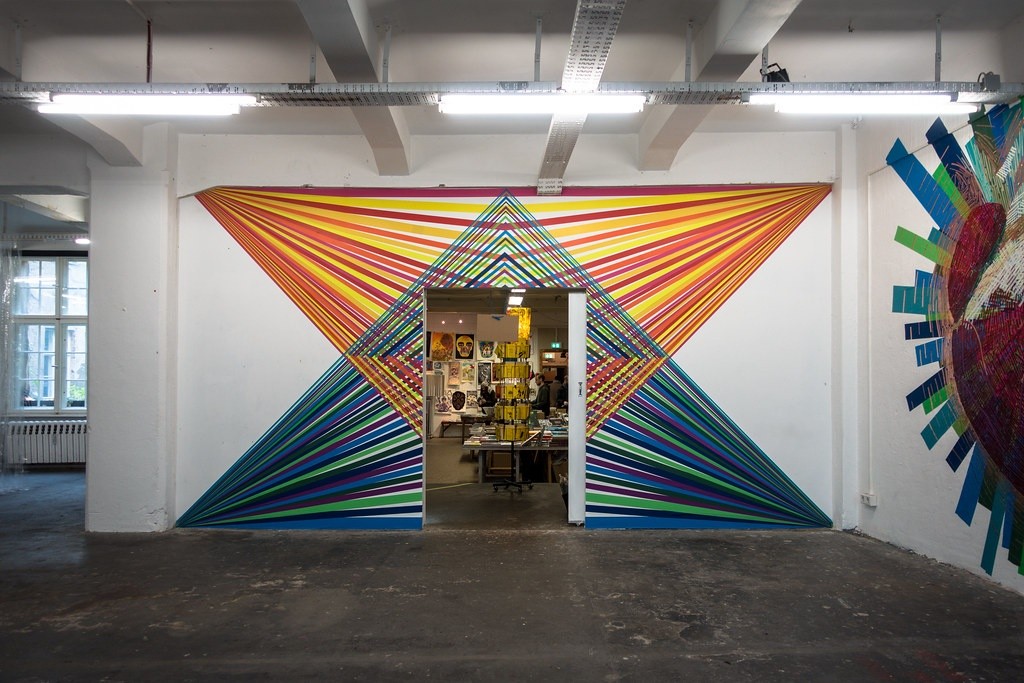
[860,492,877,508]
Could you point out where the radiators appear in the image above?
[4,419,89,466]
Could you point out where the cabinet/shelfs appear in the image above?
[538,348,569,411]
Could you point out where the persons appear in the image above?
[522,373,550,420]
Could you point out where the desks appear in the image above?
[460,413,488,445]
[441,421,474,439]
